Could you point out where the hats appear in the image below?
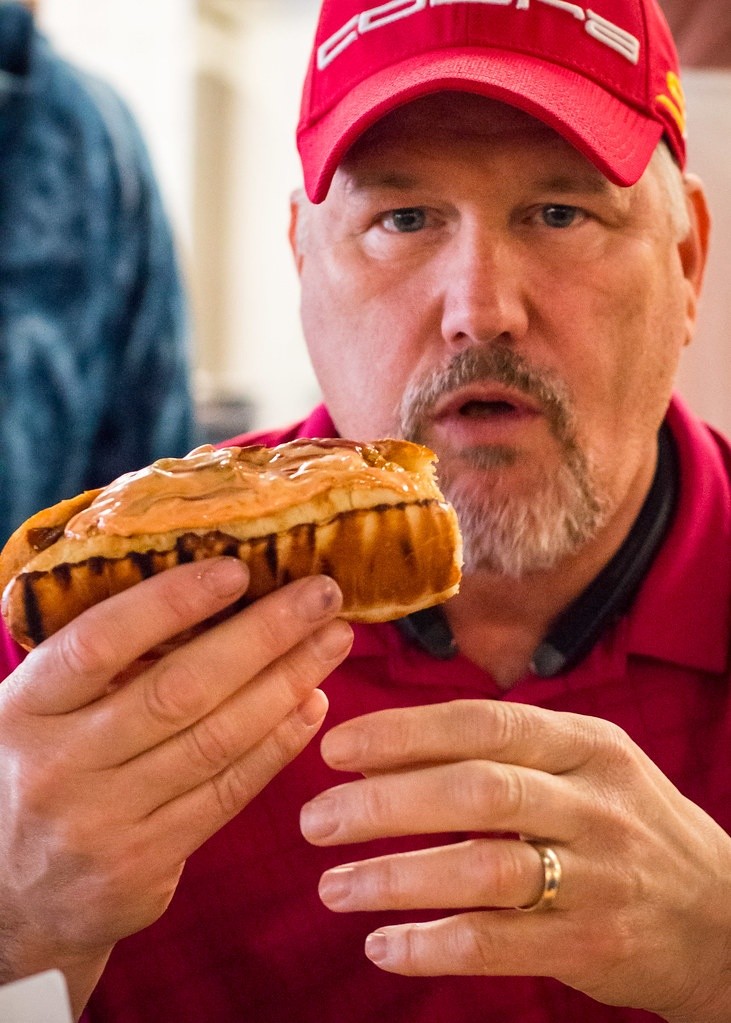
[295,0,688,206]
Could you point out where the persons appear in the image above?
[1,0,731,1023]
[1,1,194,552]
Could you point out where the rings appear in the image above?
[511,838,562,915]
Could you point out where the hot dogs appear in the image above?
[0,435,459,655]
